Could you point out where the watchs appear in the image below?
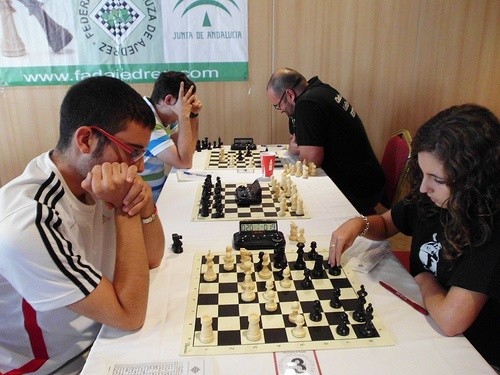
[190,112,199,118]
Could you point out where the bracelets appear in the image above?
[358,216,369,238]
[143,207,159,224]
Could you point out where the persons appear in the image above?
[267,67,390,214]
[136,71,204,204]
[327,105,500,370]
[0,76,166,375]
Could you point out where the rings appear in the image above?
[330,245,335,247]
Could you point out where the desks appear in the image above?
[79,144,500,375]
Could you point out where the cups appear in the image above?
[259,152,275,177]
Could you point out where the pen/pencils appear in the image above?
[379,280,429,315]
[184,172,207,177]
[261,144,284,147]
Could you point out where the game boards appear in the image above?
[179,244,397,354]
[204,147,285,171]
[190,180,312,224]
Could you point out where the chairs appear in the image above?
[368,130,413,216]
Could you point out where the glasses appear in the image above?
[275,88,290,110]
[90,125,146,163]
